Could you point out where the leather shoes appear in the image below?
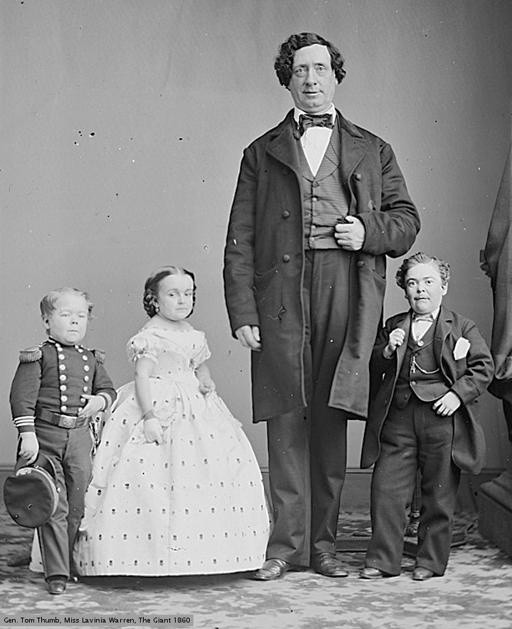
[250,559,307,581]
[44,577,70,594]
[309,551,350,578]
[412,565,435,581]
[359,566,392,579]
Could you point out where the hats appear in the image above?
[5,452,61,529]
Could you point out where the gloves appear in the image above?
[143,418,164,445]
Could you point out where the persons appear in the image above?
[9,287,119,596]
[219,31,422,583]
[26,265,274,582]
[358,252,496,580]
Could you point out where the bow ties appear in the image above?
[299,112,335,132]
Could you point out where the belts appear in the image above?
[32,407,93,430]
